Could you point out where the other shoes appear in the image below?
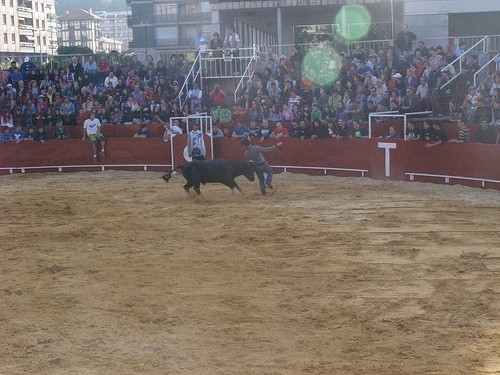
[94,157,100,163]
[262,191,266,195]
[266,183,273,189]
[100,152,106,157]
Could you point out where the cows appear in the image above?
[160,159,255,195]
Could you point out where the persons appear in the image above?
[187,121,206,161]
[0,23,500,146]
[82,111,106,158]
[240,135,284,195]
